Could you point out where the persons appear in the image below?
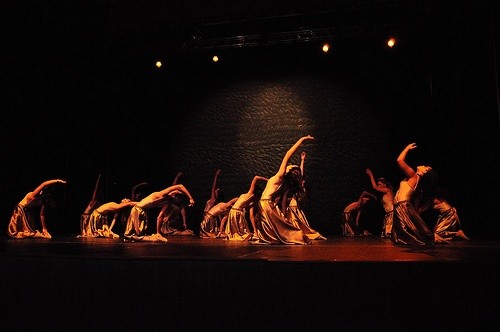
[343,190,377,236]
[124,172,197,243]
[224,175,270,242]
[87,181,150,238]
[6,178,68,238]
[199,198,245,239]
[203,169,223,221]
[253,134,315,244]
[286,149,311,230]
[429,196,470,241]
[80,173,104,236]
[366,169,395,237]
[390,141,448,248]
